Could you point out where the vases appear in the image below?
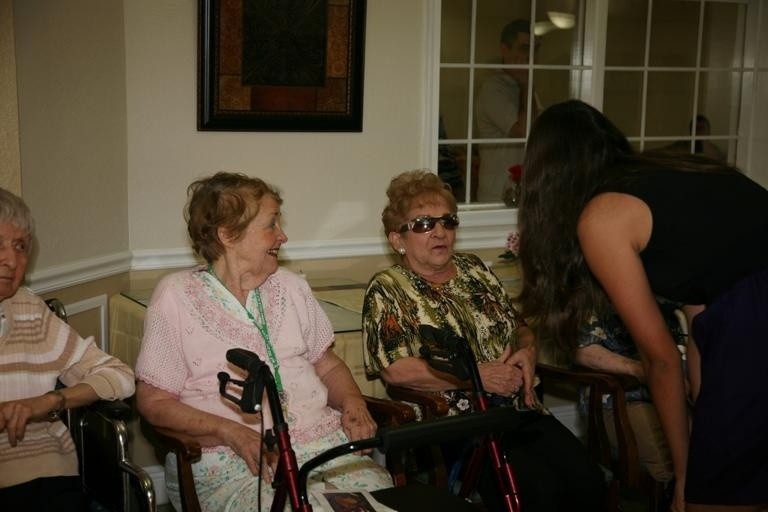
[503,185,519,207]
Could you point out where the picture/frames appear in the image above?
[196,1,368,135]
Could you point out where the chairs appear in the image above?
[142,354,666,511]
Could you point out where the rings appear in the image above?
[512,384,518,393]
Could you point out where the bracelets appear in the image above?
[44,390,66,422]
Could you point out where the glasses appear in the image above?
[397,213,459,234]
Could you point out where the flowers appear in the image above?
[508,164,521,188]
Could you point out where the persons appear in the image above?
[360,168,611,510]
[135,171,480,512]
[688,114,721,161]
[0,188,137,512]
[471,18,544,202]
[506,99,768,511]
[567,303,706,482]
